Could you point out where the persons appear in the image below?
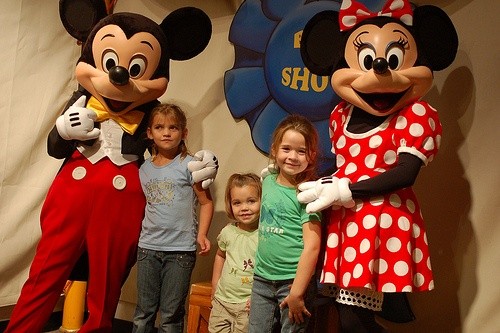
[133,103,214,333]
[247,114,321,333]
[207,172,264,333]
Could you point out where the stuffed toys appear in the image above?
[4,0,219,333]
[261,0,458,333]
